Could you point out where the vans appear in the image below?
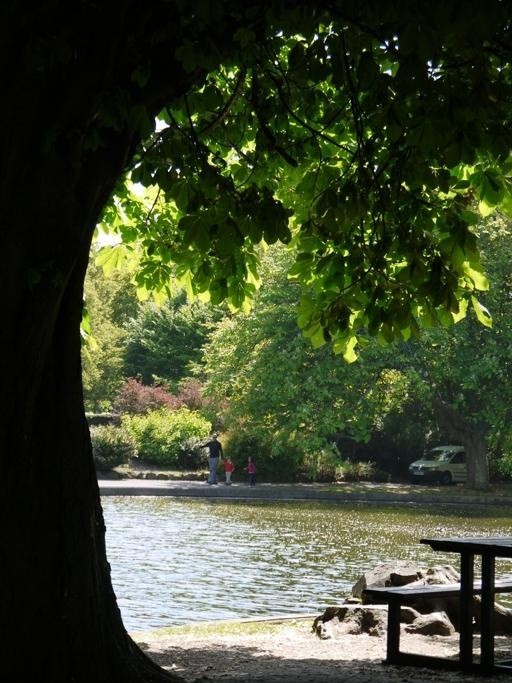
[408,445,491,486]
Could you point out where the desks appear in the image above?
[421,538,512,675]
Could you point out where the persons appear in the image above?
[199,433,224,484]
[243,455,257,487]
[223,455,235,484]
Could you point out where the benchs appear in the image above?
[363,582,512,676]
